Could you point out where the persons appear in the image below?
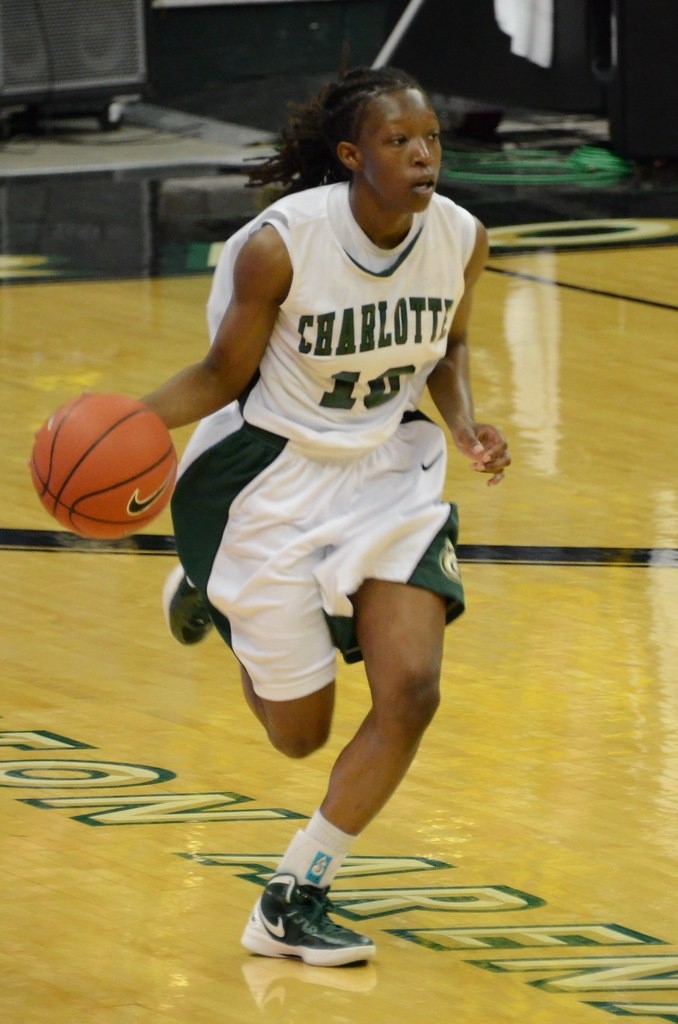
[28,63,513,970]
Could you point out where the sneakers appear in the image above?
[161,564,215,647]
[241,871,375,967]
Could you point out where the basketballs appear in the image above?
[31,390,181,541]
